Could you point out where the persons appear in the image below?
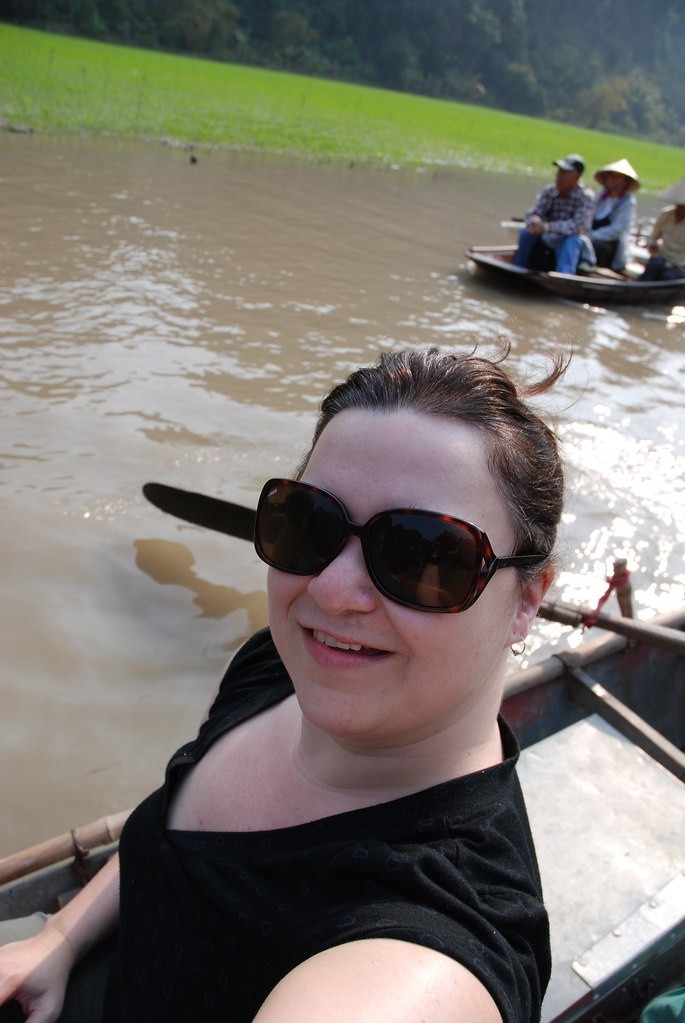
[514,155,597,275]
[639,179,685,281]
[0,349,564,1023]
[591,158,641,273]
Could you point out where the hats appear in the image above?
[594,157,640,191]
[552,154,584,173]
[657,177,685,205]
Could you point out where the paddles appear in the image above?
[141,479,685,651]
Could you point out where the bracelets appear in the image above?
[544,222,550,233]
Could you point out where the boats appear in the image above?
[1,611,685,1023]
[464,238,685,303]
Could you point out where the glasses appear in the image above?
[254,478,542,614]
